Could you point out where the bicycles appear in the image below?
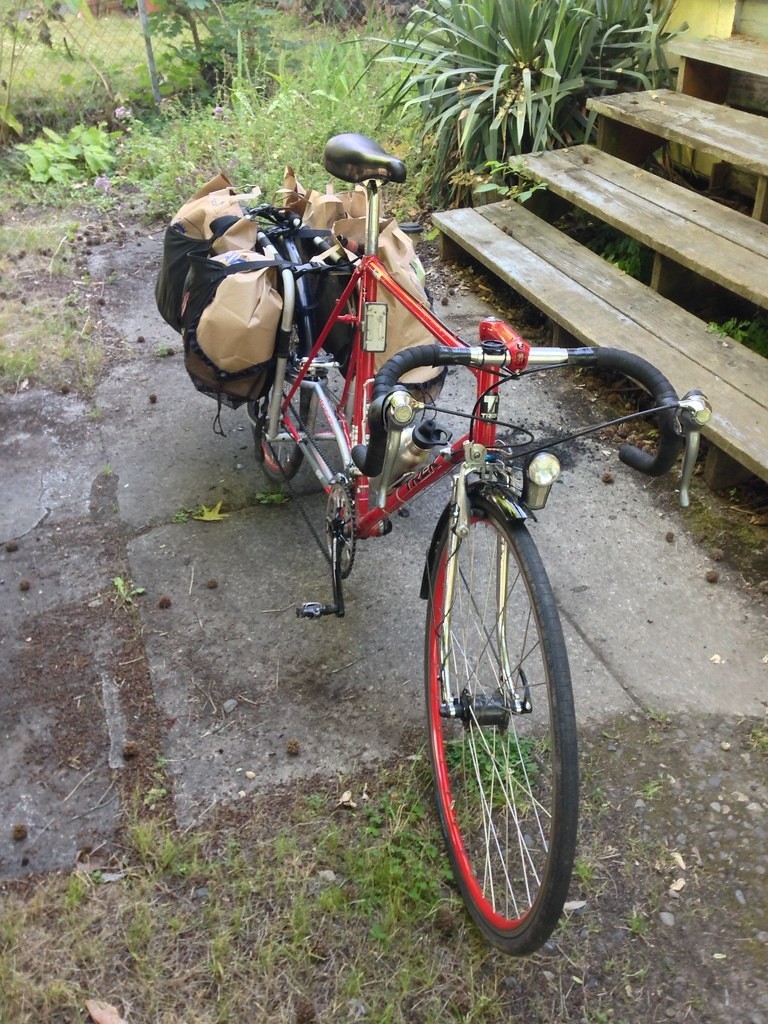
[245,134,714,958]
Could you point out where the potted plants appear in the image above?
[337,1,641,221]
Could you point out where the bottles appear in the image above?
[367,420,452,493]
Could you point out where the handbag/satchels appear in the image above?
[153,167,447,436]
